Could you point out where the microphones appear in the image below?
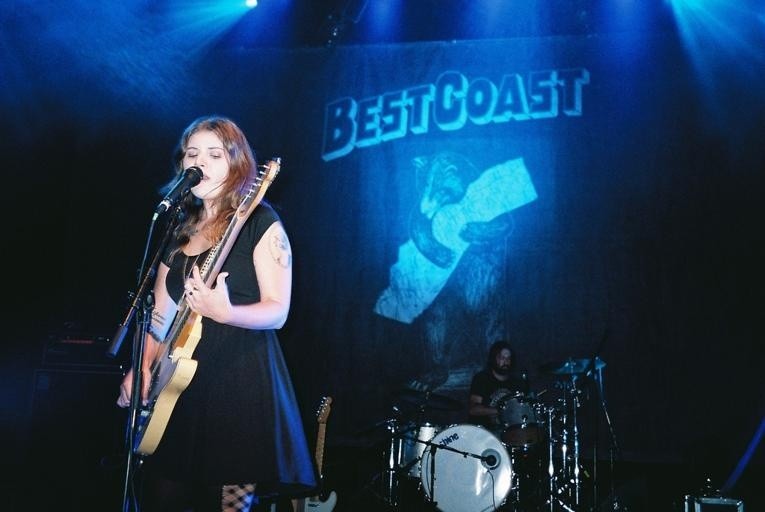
[467,453,489,461]
[155,166,203,215]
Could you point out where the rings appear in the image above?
[188,288,199,295]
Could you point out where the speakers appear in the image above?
[30,370,128,512]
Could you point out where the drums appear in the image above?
[422,419,527,512]
[404,426,436,482]
[498,398,544,444]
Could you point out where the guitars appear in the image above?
[302,397,338,512]
[125,155,283,457]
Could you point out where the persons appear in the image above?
[468,341,527,431]
[117,116,320,511]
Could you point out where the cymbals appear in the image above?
[557,358,606,377]
[395,359,451,392]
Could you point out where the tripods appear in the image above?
[543,410,575,512]
